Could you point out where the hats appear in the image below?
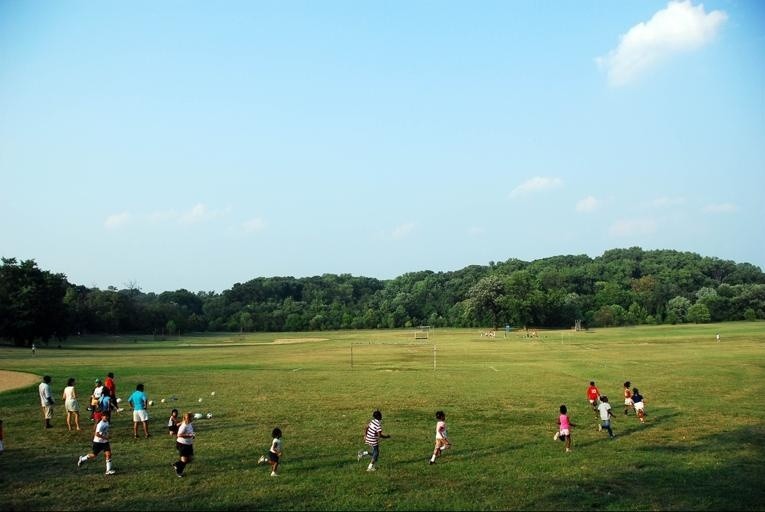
[95,379,101,383]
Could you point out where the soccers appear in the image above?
[161,399,166,403]
[195,414,201,419]
[198,398,203,403]
[207,413,213,418]
[211,392,215,396]
[149,401,154,406]
[116,397,121,403]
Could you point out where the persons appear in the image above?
[553,405,577,453]
[596,395,617,439]
[493,329,496,340]
[489,331,493,342]
[99,388,111,424]
[90,378,103,413]
[532,329,538,337]
[629,388,645,424]
[586,381,600,411]
[429,411,449,464]
[0,420,5,452]
[167,409,180,435]
[78,411,116,475]
[716,333,720,344]
[61,378,81,431]
[257,428,282,478]
[172,413,197,477]
[127,384,152,439]
[623,381,636,417]
[105,372,124,413]
[38,376,55,429]
[358,411,390,472]
[31,344,37,356]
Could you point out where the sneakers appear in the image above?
[357,450,365,463]
[565,447,574,453]
[436,447,442,457]
[270,471,280,476]
[429,460,438,465]
[104,469,116,476]
[553,431,559,441]
[598,424,603,433]
[133,434,140,439]
[365,467,378,472]
[144,434,152,439]
[77,455,84,467]
[172,461,185,478]
[116,407,124,412]
[257,454,267,465]
[594,409,646,423]
[610,434,616,440]
